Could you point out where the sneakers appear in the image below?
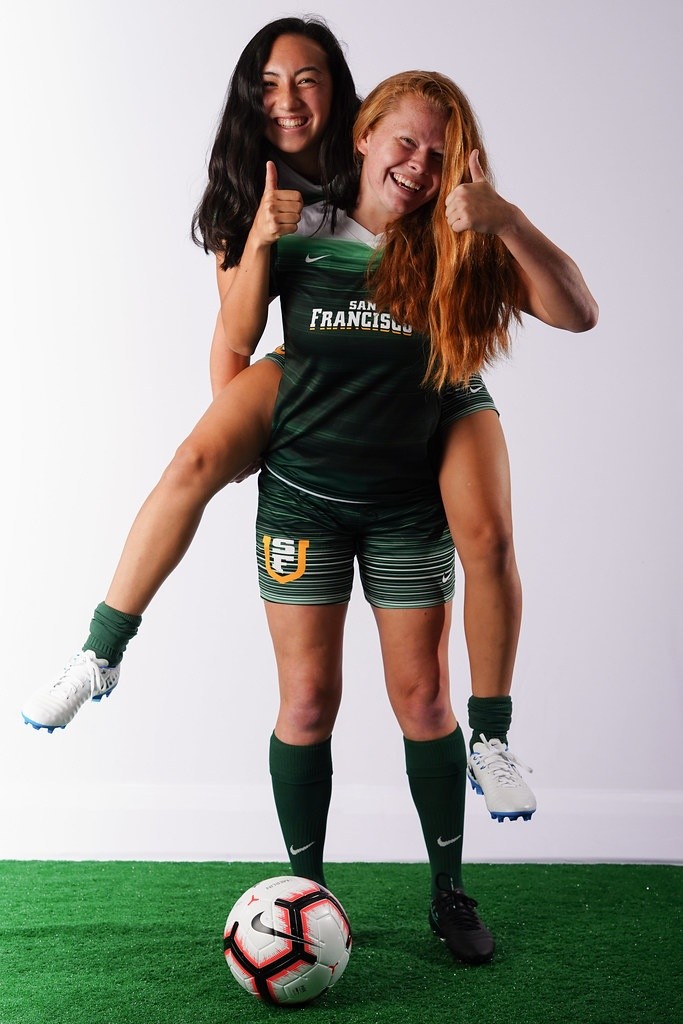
[18,650,119,735]
[428,872,495,965]
[466,733,536,823]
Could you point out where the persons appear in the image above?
[208,73,498,965]
[17,17,600,825]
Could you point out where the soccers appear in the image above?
[223,876,355,1006]
